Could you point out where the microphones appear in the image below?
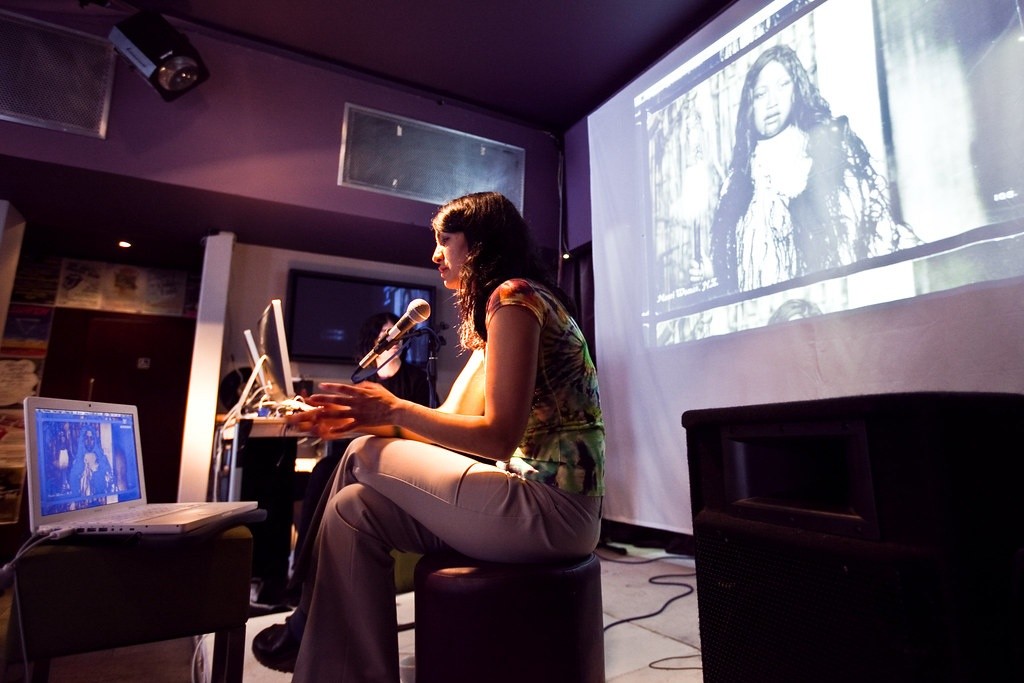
[353,298,431,376]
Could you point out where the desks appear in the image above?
[211,417,367,502]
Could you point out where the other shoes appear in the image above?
[252,622,300,675]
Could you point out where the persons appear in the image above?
[709,46,925,296]
[51,423,127,510]
[251,189,607,683]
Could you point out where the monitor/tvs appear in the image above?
[243,298,295,408]
[285,268,438,378]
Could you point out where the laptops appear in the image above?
[23,396,258,534]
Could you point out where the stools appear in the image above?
[415,552,605,683]
[0,525,252,683]
